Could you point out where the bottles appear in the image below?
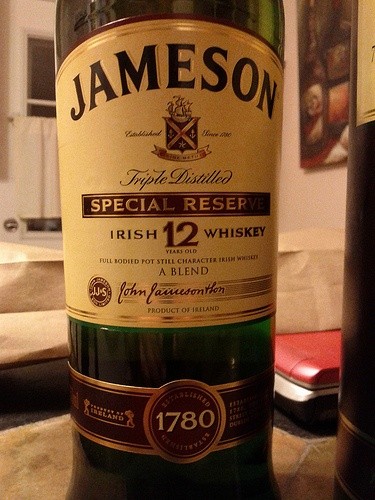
[53,1,284,499]
